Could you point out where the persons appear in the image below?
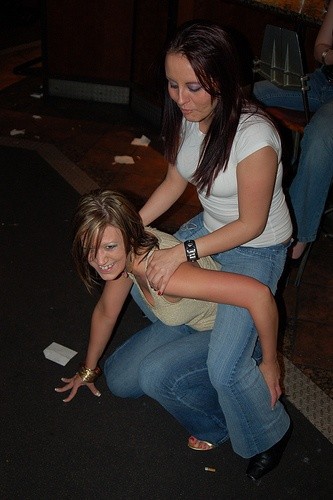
[245,0,333,264]
[137,24,292,481]
[55,191,283,450]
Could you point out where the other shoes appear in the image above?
[285,236,308,268]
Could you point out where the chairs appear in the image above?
[252,24,317,167]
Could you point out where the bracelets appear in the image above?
[322,48,331,65]
[183,239,200,262]
[78,362,100,382]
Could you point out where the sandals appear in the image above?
[188,433,216,450]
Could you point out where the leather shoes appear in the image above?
[245,435,288,482]
